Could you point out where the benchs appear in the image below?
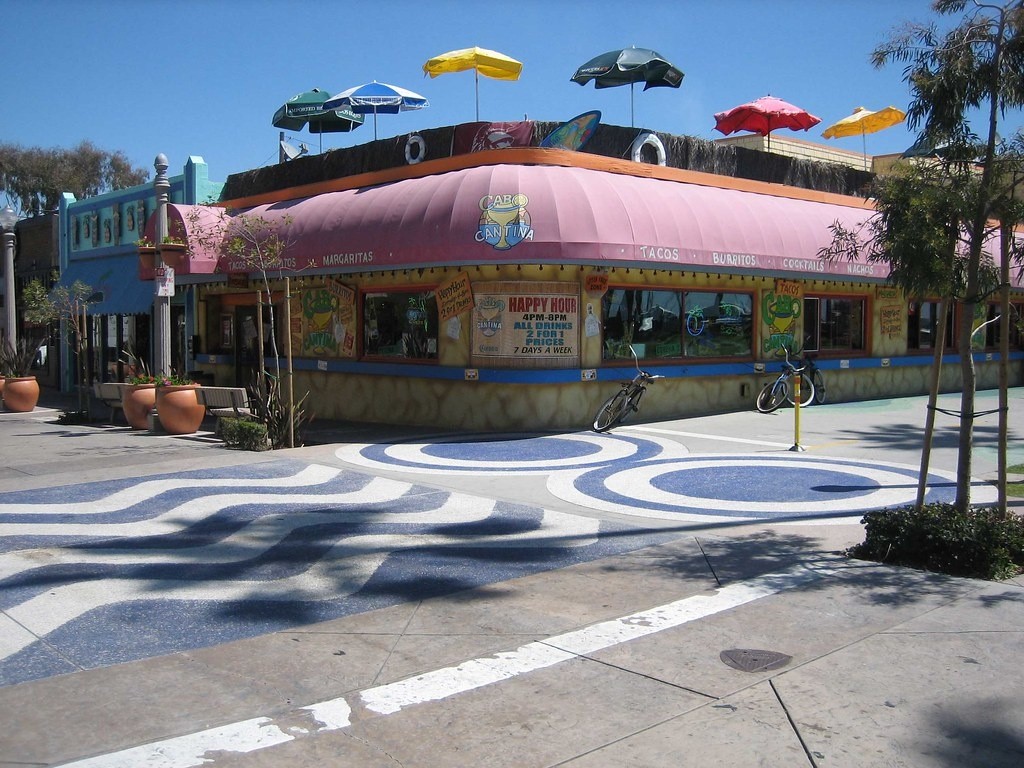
[94,382,135,426]
[194,386,258,417]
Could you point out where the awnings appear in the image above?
[140,163,1024,287]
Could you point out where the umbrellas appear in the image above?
[822,107,905,183]
[569,45,685,159]
[421,47,524,121]
[272,89,365,155]
[323,80,430,140]
[712,96,820,185]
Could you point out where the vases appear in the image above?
[154,384,206,435]
[138,247,155,269]
[160,244,186,266]
[122,384,156,430]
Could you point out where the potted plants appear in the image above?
[0,335,47,412]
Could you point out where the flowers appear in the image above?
[130,372,198,387]
[133,236,154,247]
[162,236,183,244]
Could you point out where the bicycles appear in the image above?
[592,345,666,433]
[756,335,827,414]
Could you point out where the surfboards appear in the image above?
[540,109,602,151]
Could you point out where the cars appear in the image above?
[605,306,680,343]
[682,302,752,336]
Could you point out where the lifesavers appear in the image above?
[405,137,425,164]
[631,133,666,166]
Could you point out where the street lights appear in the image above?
[0,204,23,379]
